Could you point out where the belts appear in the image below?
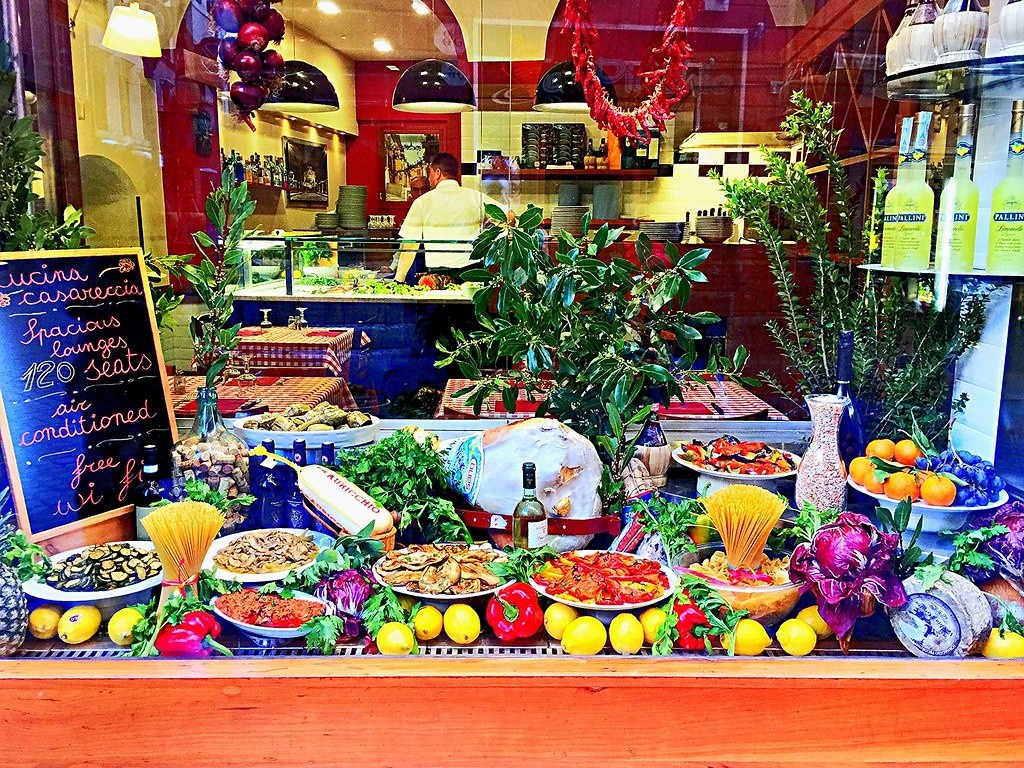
[427,266,449,272]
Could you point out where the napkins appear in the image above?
[656,401,713,415]
[226,376,279,387]
[183,398,247,412]
[306,330,344,339]
[238,330,268,338]
[495,400,543,412]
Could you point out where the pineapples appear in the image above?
[0,485,28,657]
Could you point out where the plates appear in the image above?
[695,216,734,242]
[843,476,1009,533]
[549,205,591,237]
[526,545,678,623]
[638,221,686,244]
[367,542,517,605]
[21,540,162,623]
[672,441,802,502]
[199,525,340,584]
[315,184,368,230]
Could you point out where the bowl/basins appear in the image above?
[669,547,816,629]
[303,267,338,281]
[252,265,280,280]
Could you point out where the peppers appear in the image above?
[154,611,235,659]
[486,582,543,642]
[688,482,720,545]
[559,0,703,150]
[672,588,717,656]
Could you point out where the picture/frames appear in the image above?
[282,136,329,206]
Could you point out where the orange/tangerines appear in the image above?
[849,438,955,506]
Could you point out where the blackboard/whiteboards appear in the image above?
[0,247,182,543]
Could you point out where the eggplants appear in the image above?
[43,543,161,592]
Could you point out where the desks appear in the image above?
[167,375,360,417]
[433,381,789,421]
[190,326,380,414]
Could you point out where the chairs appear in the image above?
[189,310,223,342]
[352,312,383,412]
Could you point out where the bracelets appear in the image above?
[394,278,406,285]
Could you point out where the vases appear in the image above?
[794,394,850,516]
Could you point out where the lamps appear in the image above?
[102,2,162,58]
[532,59,618,113]
[391,1,476,114]
[262,8,339,113]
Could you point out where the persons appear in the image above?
[390,153,508,289]
[380,176,430,286]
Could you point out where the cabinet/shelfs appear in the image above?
[218,233,500,419]
[855,55,1024,284]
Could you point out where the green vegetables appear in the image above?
[0,529,54,585]
[126,594,218,659]
[298,583,420,654]
[294,273,432,293]
[148,429,705,596]
[768,492,1024,641]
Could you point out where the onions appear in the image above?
[212,0,286,121]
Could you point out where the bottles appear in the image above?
[235,437,339,538]
[132,444,166,540]
[987,98,1023,277]
[511,461,548,552]
[880,109,934,271]
[935,103,979,271]
[831,327,866,513]
[217,147,287,188]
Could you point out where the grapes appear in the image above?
[915,448,1004,507]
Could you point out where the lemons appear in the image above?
[542,603,667,655]
[377,604,480,654]
[29,603,148,645]
[293,269,301,278]
[720,605,833,656]
[980,628,1024,659]
